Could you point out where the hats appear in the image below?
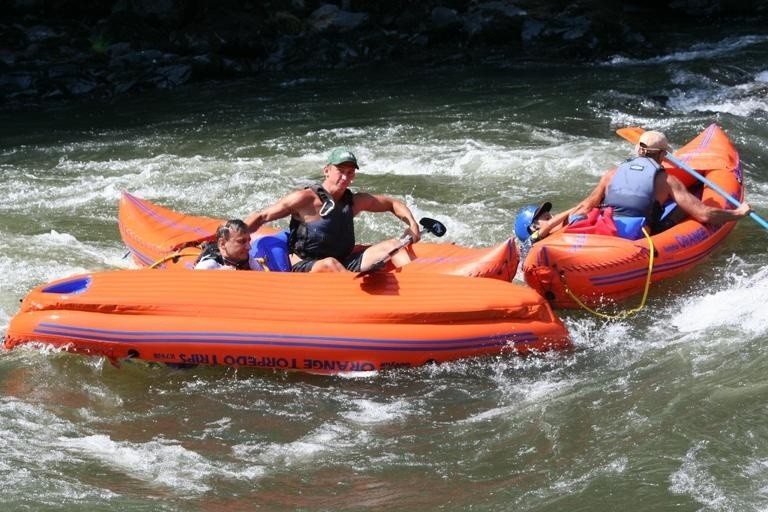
[326,148,359,169]
[638,130,673,153]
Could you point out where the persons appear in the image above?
[549,129,756,236]
[242,146,422,273]
[195,218,268,271]
[512,200,586,244]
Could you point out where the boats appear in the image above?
[522,119,745,311]
[113,191,521,288]
[4,270,573,371]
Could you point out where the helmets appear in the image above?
[515,201,552,241]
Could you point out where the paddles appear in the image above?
[615,127,768,228]
[354,218,447,281]
